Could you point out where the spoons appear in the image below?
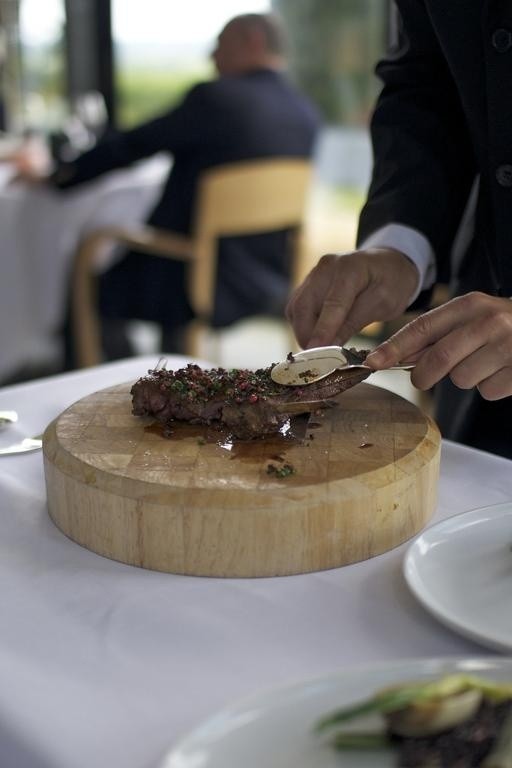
[270,345,418,386]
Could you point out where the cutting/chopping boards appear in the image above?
[41,368,442,578]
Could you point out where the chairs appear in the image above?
[71,159,310,366]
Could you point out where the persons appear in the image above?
[280,1,512,459]
[12,7,321,379]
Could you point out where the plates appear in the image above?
[166,653,512,768]
[403,501,512,653]
